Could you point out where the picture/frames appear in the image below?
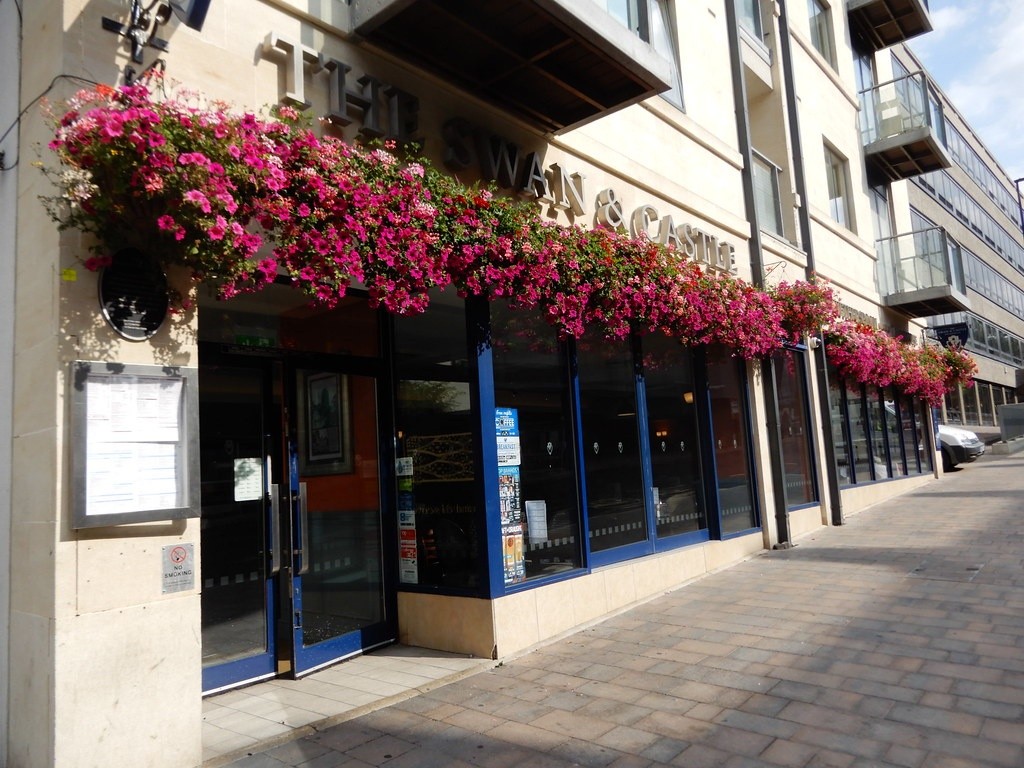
[295,365,354,475]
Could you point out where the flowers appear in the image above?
[30,67,978,406]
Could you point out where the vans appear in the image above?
[824,400,985,470]
